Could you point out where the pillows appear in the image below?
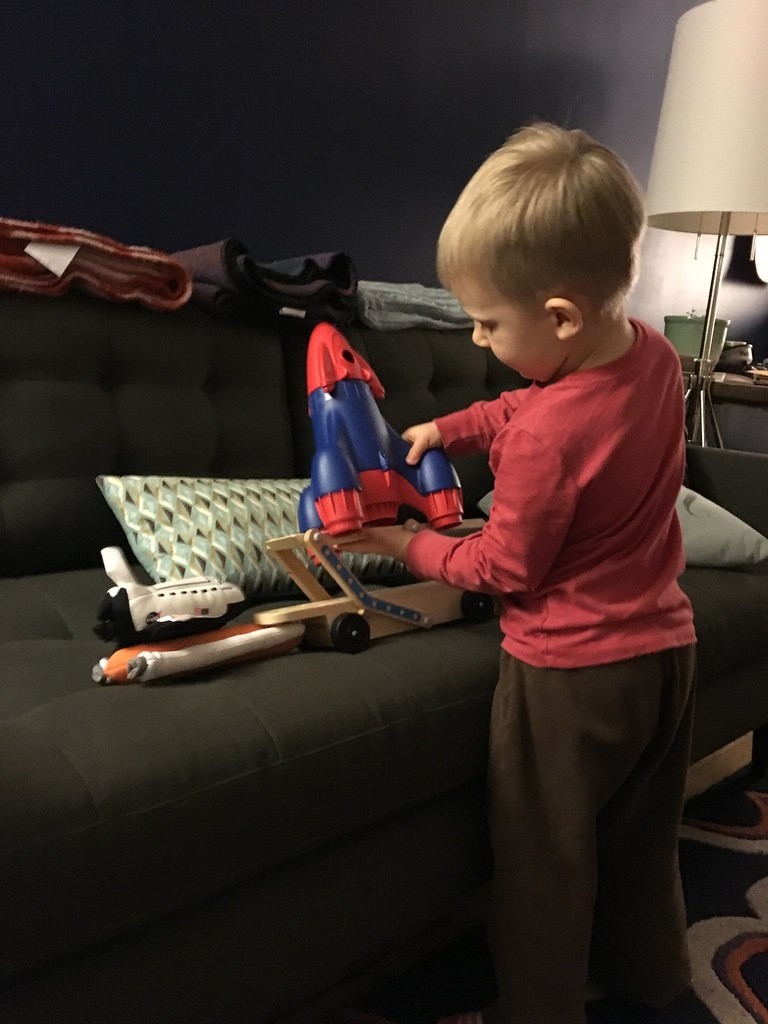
[94,475,411,608]
[475,481,768,568]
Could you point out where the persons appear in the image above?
[334,121,698,1024]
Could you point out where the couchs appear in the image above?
[0,271,767,1024]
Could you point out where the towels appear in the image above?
[0,218,477,333]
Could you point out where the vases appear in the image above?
[660,315,732,374]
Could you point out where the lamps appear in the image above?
[640,0,768,450]
